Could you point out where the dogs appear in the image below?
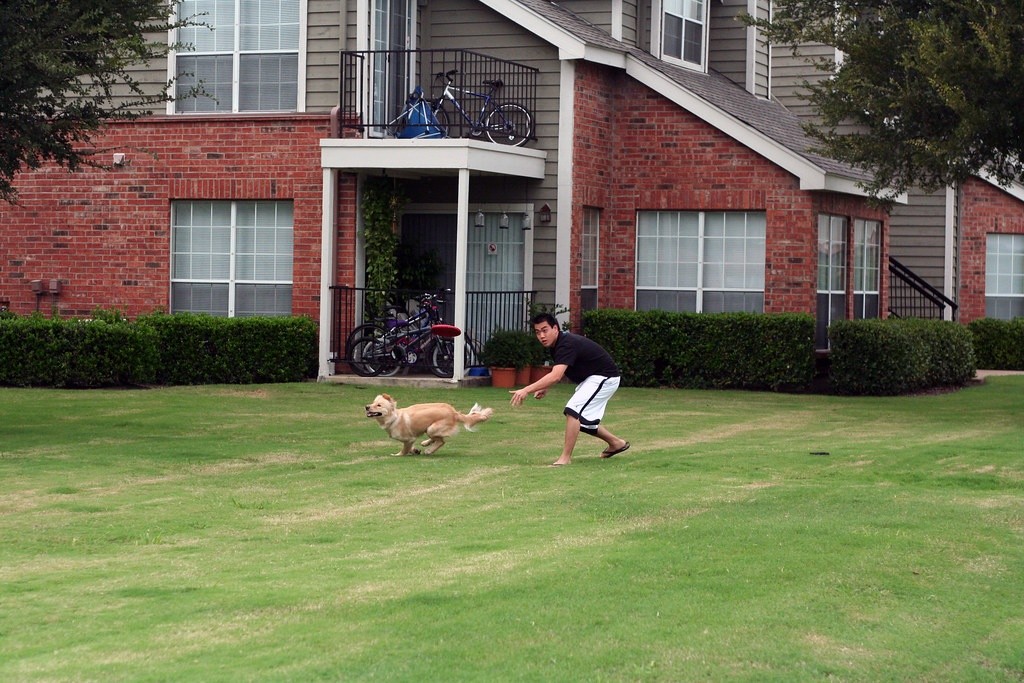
[365,393,494,456]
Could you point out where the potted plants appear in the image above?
[479,324,553,388]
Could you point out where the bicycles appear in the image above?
[401,69,534,146]
[344,287,476,378]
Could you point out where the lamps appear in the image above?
[474,208,487,227]
[521,215,531,231]
[499,209,509,230]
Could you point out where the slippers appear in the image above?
[548,463,563,468]
[602,442,630,458]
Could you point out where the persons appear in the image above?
[509,313,631,468]
